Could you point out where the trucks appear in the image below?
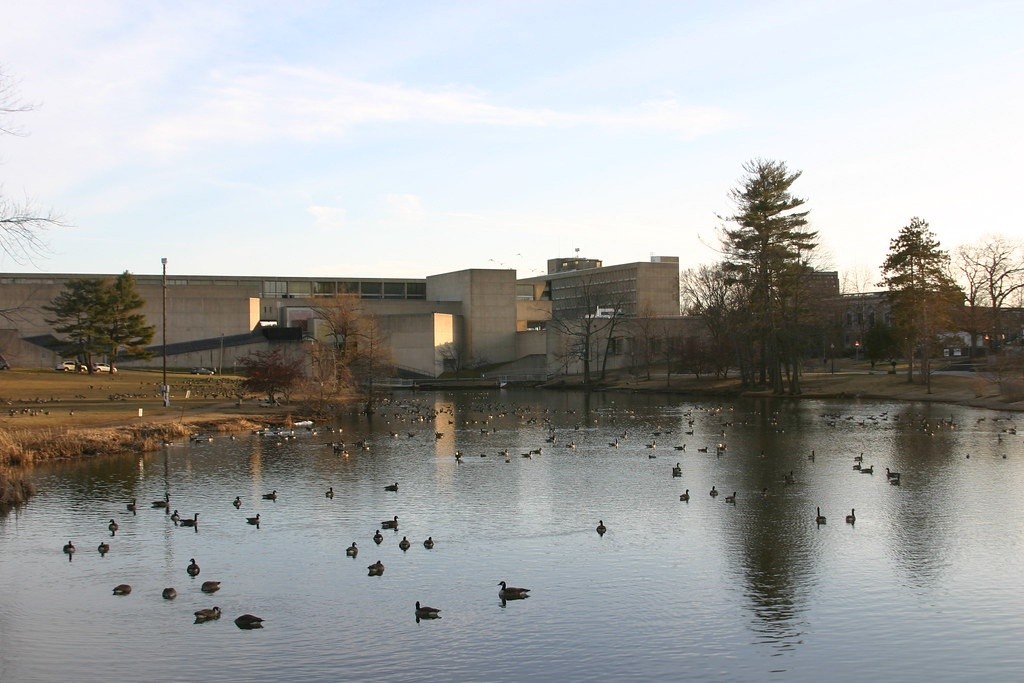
[584,305,623,319]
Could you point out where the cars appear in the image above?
[190,366,215,375]
[92,363,118,373]
[55,360,87,372]
[0,353,11,370]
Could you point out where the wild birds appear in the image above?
[162,558,265,630]
[171,510,200,525]
[108,519,118,531]
[112,584,132,595]
[415,580,531,618]
[97,542,109,553]
[233,490,278,525]
[180,523,198,534]
[63,540,75,553]
[346,516,434,576]
[644,405,1017,525]
[498,594,531,609]
[151,491,172,507]
[596,520,606,534]
[384,483,399,491]
[127,500,136,511]
[415,617,442,626]
[6,378,280,416]
[190,392,629,463]
[325,487,334,498]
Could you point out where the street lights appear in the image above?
[161,257,168,406]
